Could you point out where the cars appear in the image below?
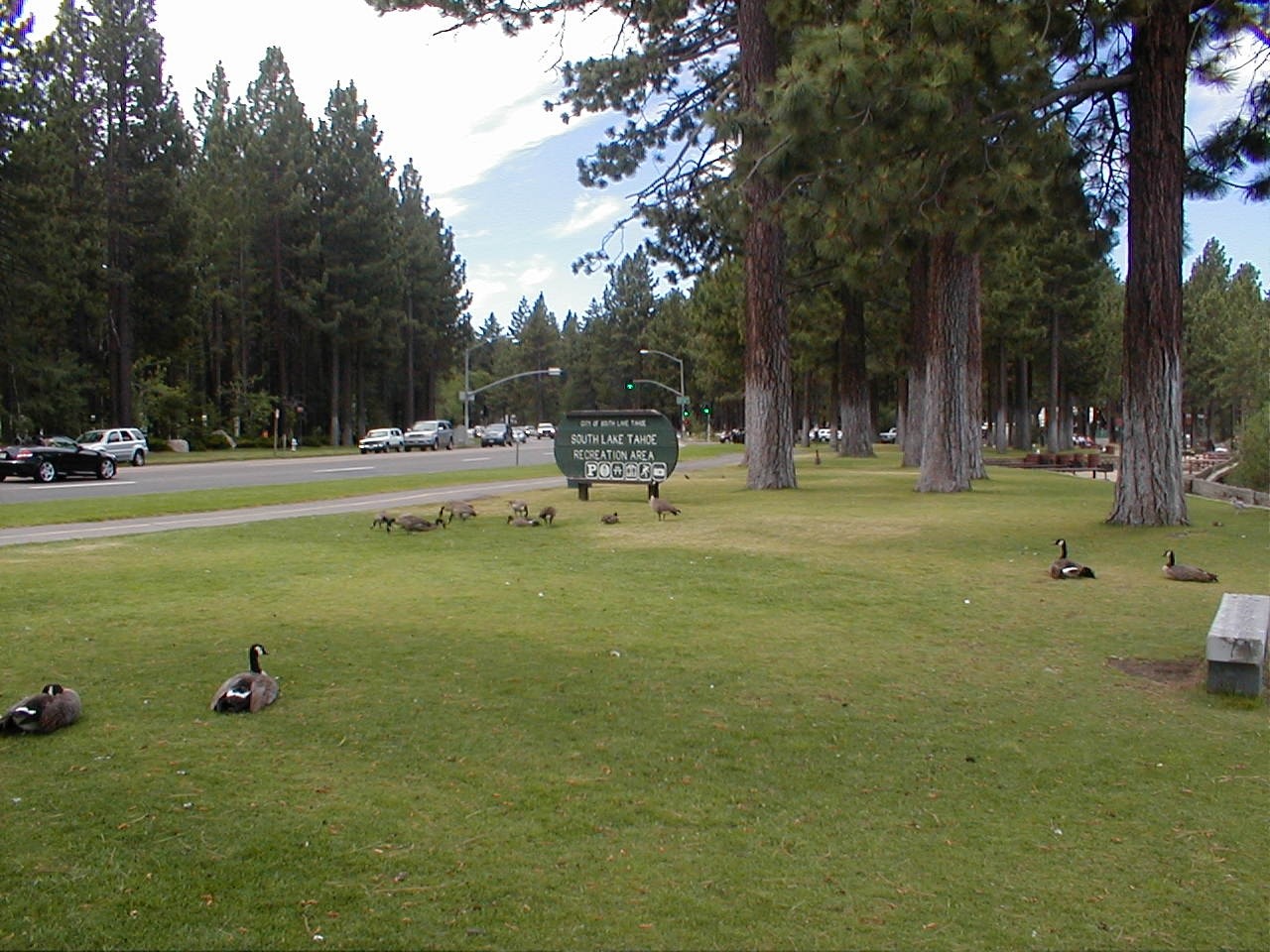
[879,427,897,444]
[481,423,512,447]
[719,428,745,444]
[473,425,485,438]
[510,424,538,444]
[808,421,843,443]
[0,435,117,484]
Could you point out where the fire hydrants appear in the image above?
[291,438,298,453]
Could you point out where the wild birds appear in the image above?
[1230,497,1249,514]
[650,494,681,521]
[369,500,556,532]
[601,512,620,525]
[0,684,81,733]
[1052,539,1095,581]
[683,474,690,480]
[1160,548,1220,583]
[209,643,279,713]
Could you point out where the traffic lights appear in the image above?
[626,378,634,390]
[704,405,709,413]
[684,411,689,416]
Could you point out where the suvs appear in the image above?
[537,422,556,440]
[76,427,148,467]
[403,419,454,452]
[358,427,405,454]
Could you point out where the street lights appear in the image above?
[640,349,685,434]
[464,334,517,429]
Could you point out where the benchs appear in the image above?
[1207,593,1270,699]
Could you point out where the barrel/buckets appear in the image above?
[1025,453,1099,467]
